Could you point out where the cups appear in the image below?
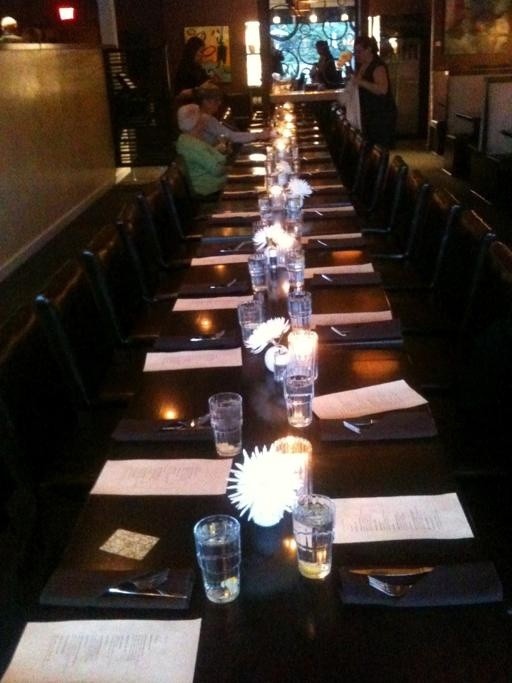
[248,254,268,292]
[283,368,315,429]
[286,250,305,288]
[287,291,312,335]
[208,392,244,458]
[193,514,242,604]
[258,107,303,234]
[292,494,336,579]
[237,302,263,347]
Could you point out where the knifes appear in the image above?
[348,566,434,576]
[108,587,188,600]
[160,425,211,433]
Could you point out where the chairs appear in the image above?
[353,158,408,217]
[140,184,198,252]
[352,148,386,213]
[85,225,174,341]
[404,244,512,389]
[162,158,214,221]
[117,202,193,279]
[320,101,370,191]
[371,188,463,286]
[0,301,122,495]
[389,212,494,334]
[38,262,147,411]
[362,169,431,256]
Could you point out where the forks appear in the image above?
[366,574,418,599]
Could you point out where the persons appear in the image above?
[175,36,218,88]
[326,89,327,90]
[195,80,271,144]
[314,39,339,90]
[351,35,398,150]
[0,16,24,42]
[175,103,241,204]
